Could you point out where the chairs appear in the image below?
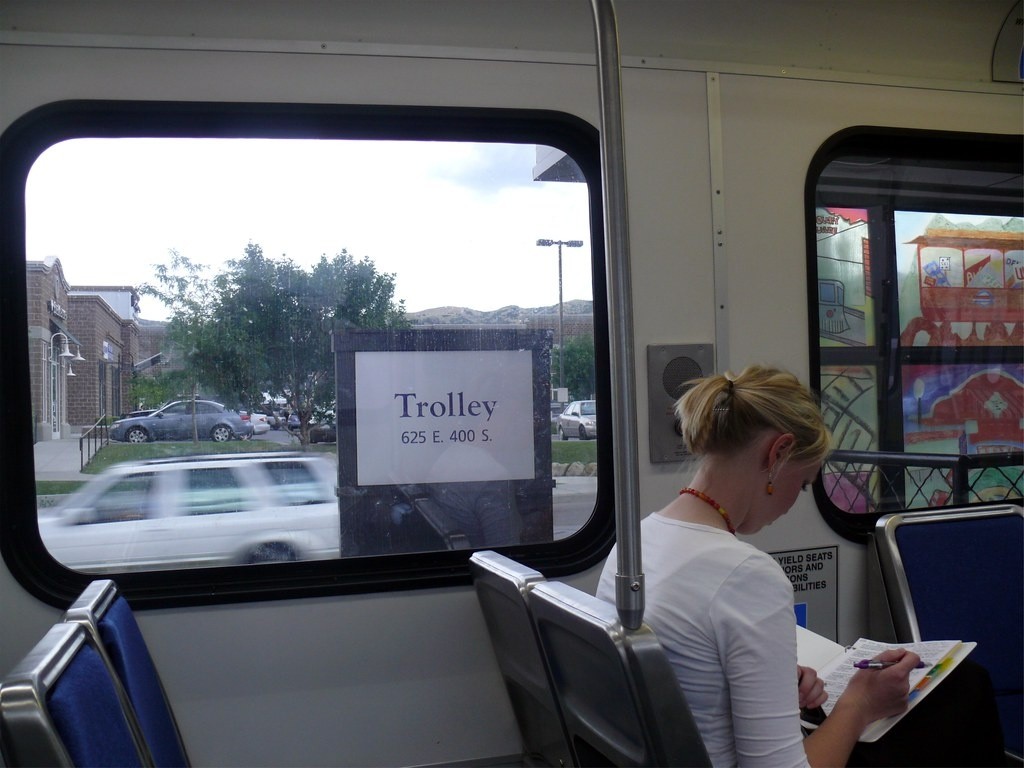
[62,580,193,768]
[875,504,1024,768]
[528,580,713,768]
[0,623,148,768]
[470,550,576,768]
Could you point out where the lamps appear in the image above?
[47,333,86,376]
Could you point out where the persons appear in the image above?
[595,364,1005,768]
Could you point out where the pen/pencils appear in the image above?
[850,658,931,670]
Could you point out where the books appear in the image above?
[791,623,978,743]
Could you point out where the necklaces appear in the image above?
[678,486,735,536]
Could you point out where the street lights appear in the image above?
[537,238,585,403]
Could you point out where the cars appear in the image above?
[267,409,336,443]
[234,408,270,441]
[555,400,597,441]
[108,400,254,445]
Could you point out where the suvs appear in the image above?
[32,453,347,568]
